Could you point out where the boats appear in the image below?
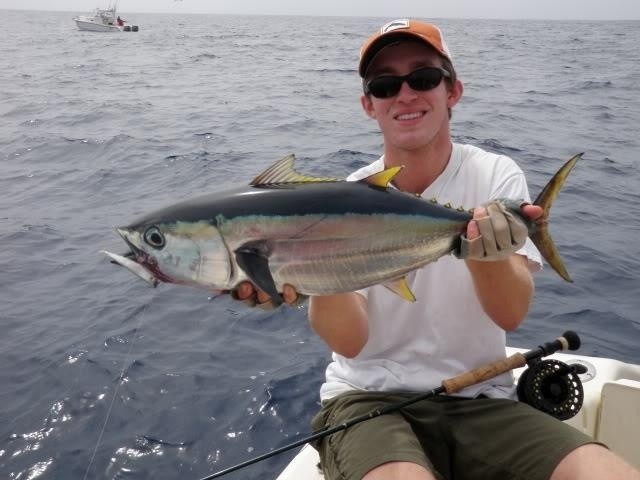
[74,1,139,32]
[270,343,640,479]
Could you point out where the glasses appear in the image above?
[362,66,452,98]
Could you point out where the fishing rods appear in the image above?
[199,330,589,480]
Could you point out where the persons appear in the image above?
[115,15,126,27]
[226,17,639,480]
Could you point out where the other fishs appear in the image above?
[99,152,585,304]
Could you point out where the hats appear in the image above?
[359,18,453,79]
